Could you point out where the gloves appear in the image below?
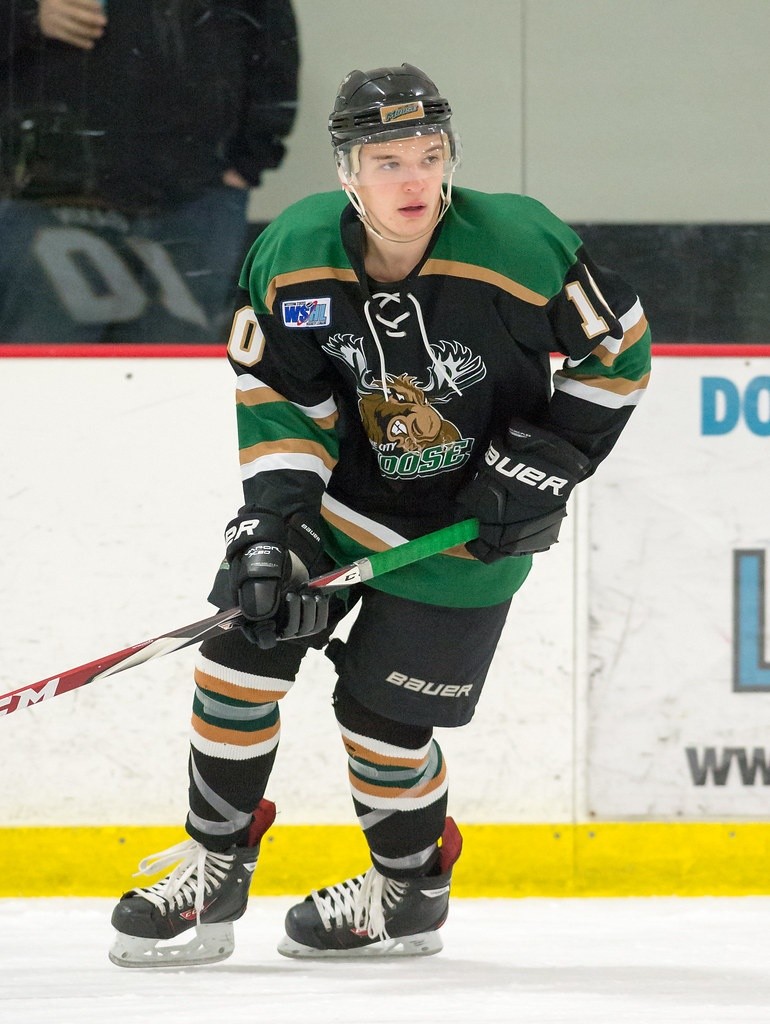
[465,430,578,562]
[226,505,332,648]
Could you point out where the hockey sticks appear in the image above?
[0,517,483,720]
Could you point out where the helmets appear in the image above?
[328,65,458,155]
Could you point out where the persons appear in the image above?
[110,64,651,968]
[0,0,300,346]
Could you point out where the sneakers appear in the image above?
[278,819,462,959]
[108,803,274,970]
